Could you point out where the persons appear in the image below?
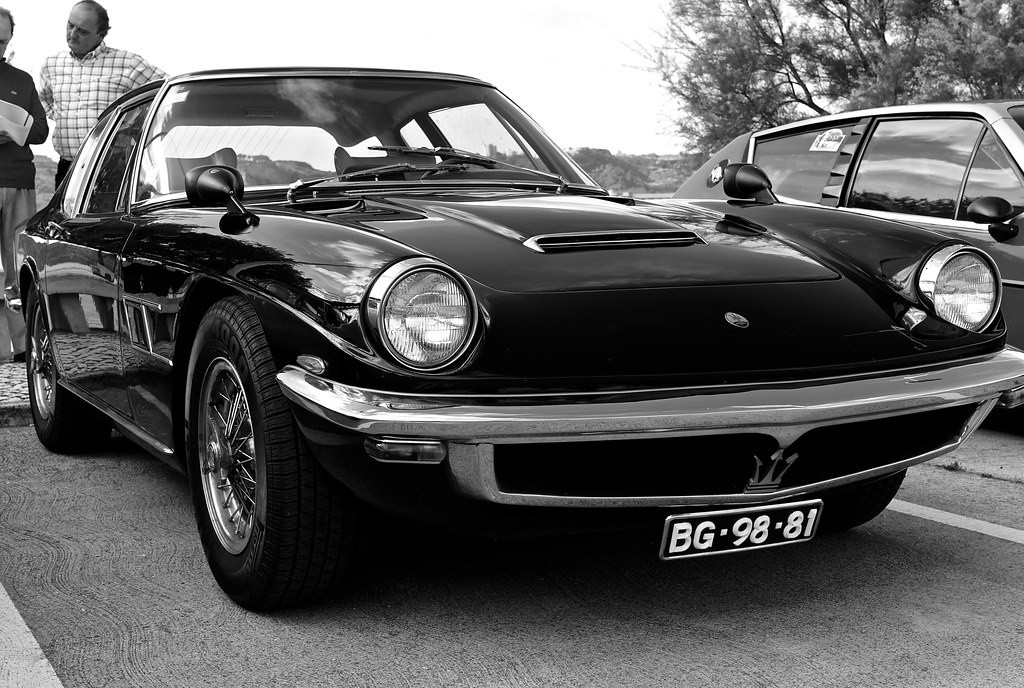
[0,5,49,362]
[37,1,172,193]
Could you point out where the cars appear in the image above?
[3,66,1024,615]
[670,98,1024,353]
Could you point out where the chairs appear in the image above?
[151,149,238,194]
[335,146,437,175]
